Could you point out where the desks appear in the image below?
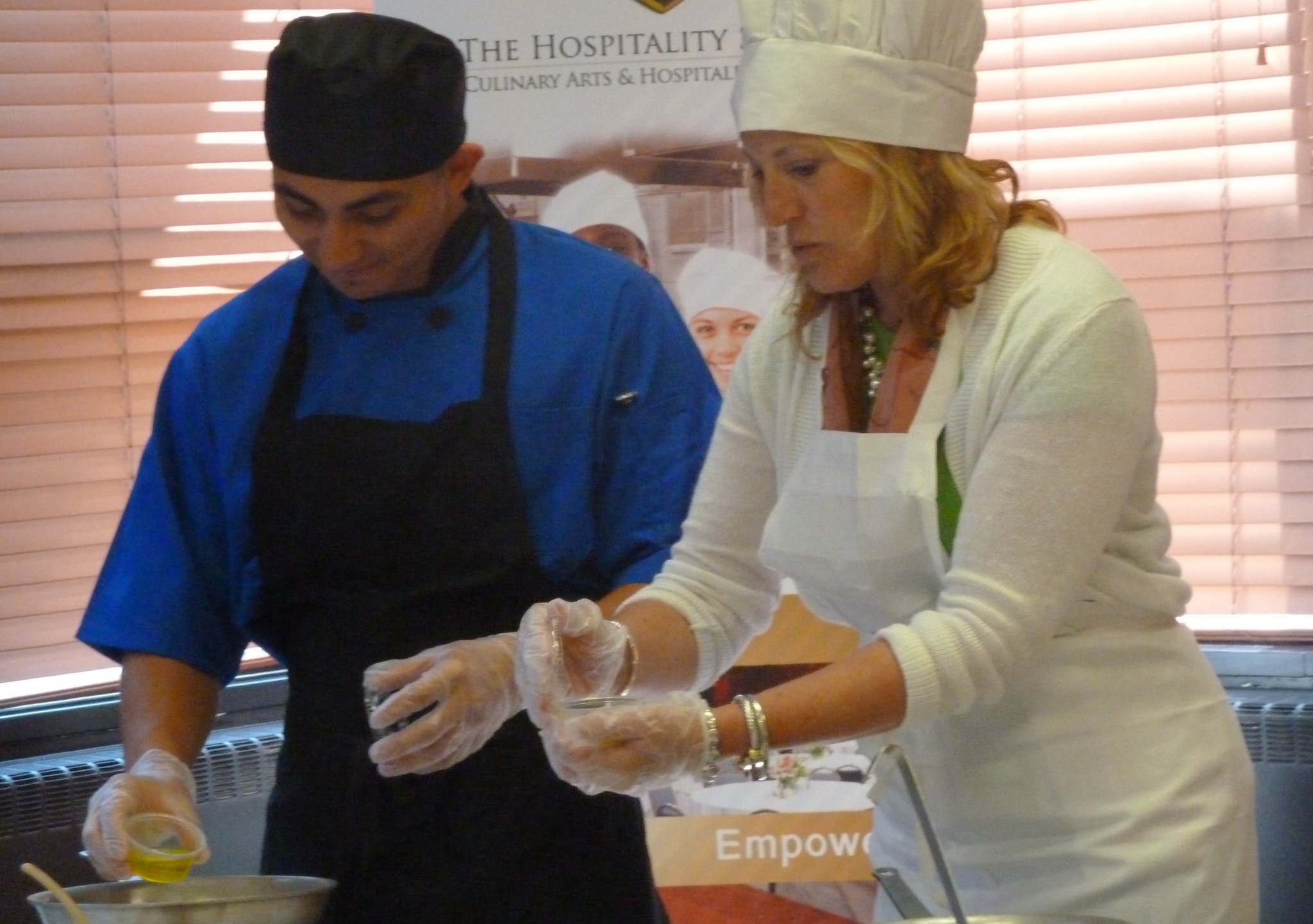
[647,879,872,924]
[676,779,871,875]
[769,749,878,772]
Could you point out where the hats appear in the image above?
[540,171,655,273]
[732,0,989,155]
[267,15,467,181]
[674,249,783,326]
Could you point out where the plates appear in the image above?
[27,876,339,923]
[694,739,874,814]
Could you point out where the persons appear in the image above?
[533,167,653,273]
[673,245,785,389]
[67,9,724,922]
[518,0,1284,924]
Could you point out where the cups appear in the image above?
[120,813,206,885]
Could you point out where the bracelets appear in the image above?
[608,616,640,697]
[692,695,720,791]
[732,692,772,783]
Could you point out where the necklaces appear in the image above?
[840,289,900,400]
